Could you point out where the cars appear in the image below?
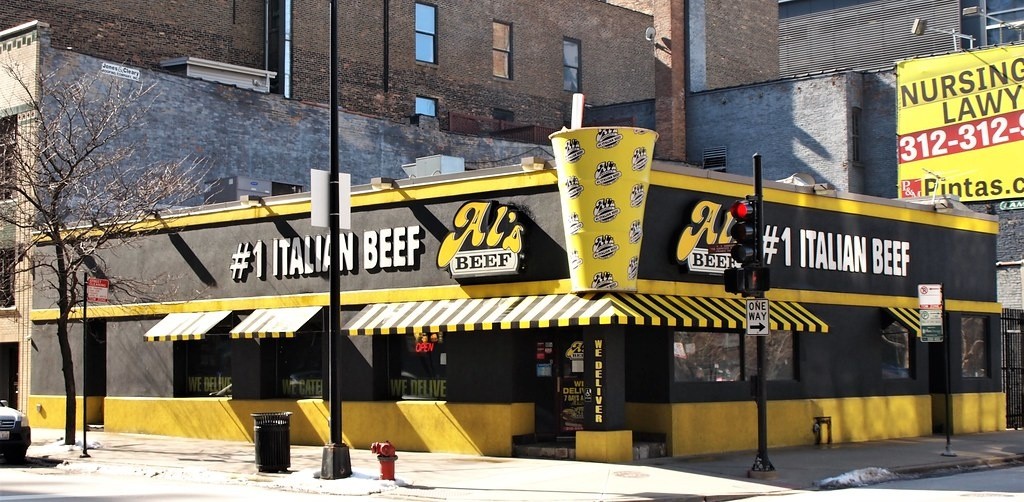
[0,399,30,460]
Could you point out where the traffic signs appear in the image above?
[746,299,770,335]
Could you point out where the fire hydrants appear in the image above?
[371,440,398,480]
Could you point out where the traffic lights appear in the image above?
[729,199,758,263]
[735,265,771,294]
